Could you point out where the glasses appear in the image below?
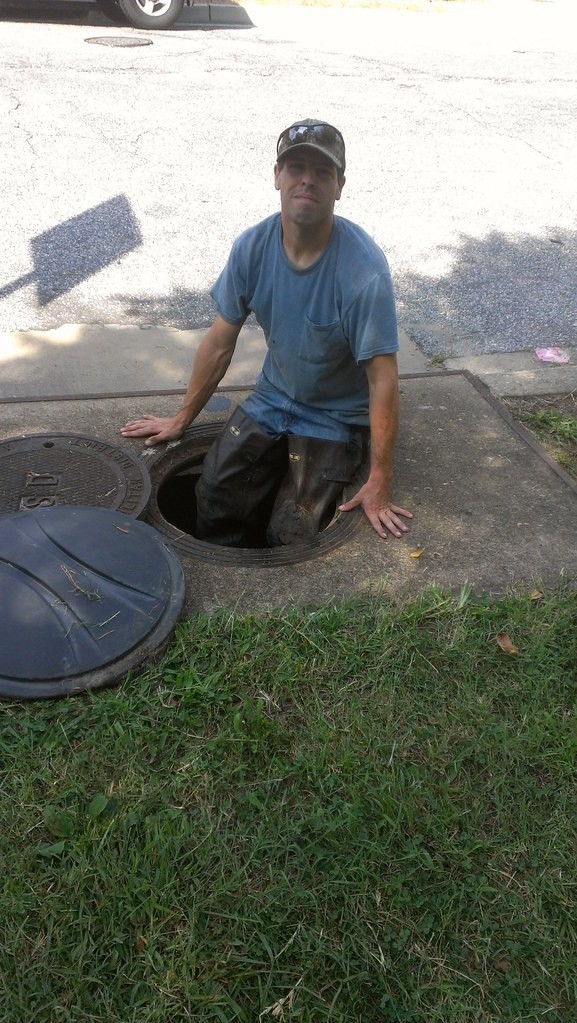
[276,124,345,153]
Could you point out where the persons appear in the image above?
[121,118,417,552]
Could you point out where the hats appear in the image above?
[276,118,346,174]
